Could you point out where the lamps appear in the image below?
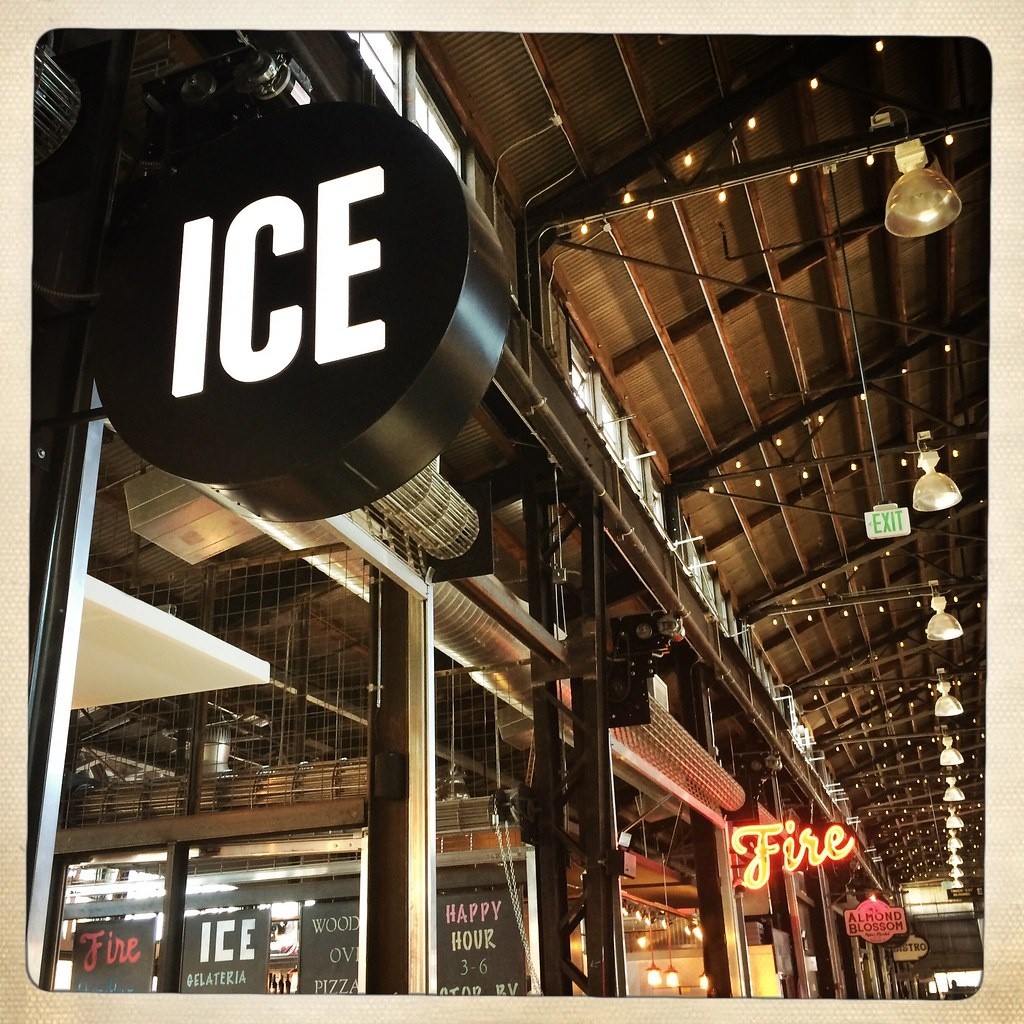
[934,680,964,717]
[926,596,963,641]
[947,830,965,889]
[623,900,708,990]
[912,451,962,511]
[940,735,965,765]
[946,806,964,829]
[884,137,962,239]
[943,776,965,801]
